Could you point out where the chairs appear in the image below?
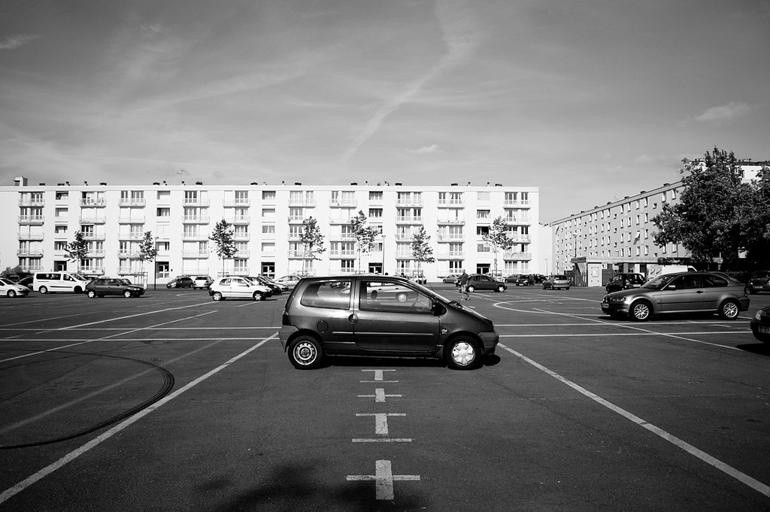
[371,290,382,308]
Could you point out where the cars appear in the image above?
[275,272,505,374]
[603,264,770,293]
[749,304,770,345]
[0,269,146,299]
[600,271,752,322]
[165,274,306,303]
[333,270,574,299]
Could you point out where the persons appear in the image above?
[457,269,470,301]
[622,278,633,290]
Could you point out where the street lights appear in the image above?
[572,233,578,257]
[556,260,560,275]
[153,236,160,291]
